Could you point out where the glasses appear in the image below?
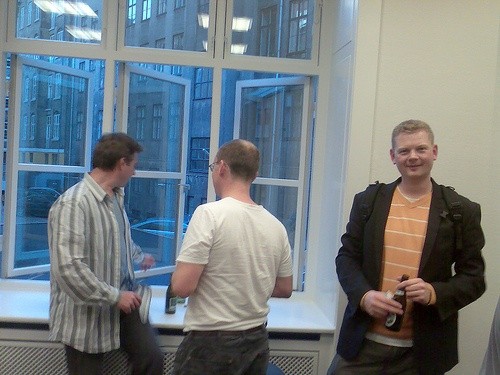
[208,161,220,172]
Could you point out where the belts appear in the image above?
[187,323,265,336]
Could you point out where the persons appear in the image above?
[170,138,293,375]
[49,132,163,375]
[326,120,487,375]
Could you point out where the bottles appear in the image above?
[384,273,410,334]
[166,283,177,315]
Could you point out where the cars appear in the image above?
[130,218,189,242]
[1,180,62,280]
